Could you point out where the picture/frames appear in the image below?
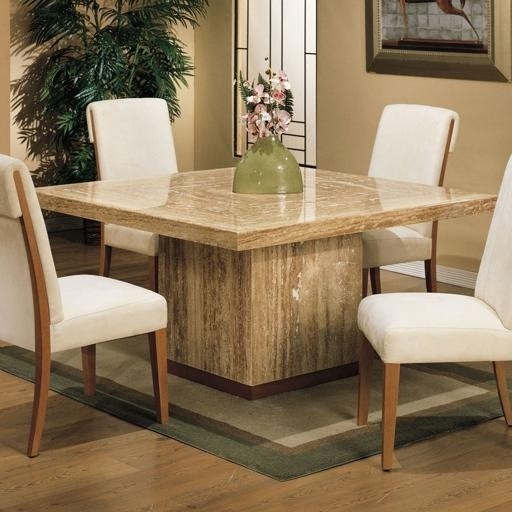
[364,0,512,83]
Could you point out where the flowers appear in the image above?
[238,54,295,141]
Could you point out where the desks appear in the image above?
[29,166,501,401]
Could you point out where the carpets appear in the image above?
[1,259,511,484]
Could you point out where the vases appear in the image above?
[232,137,305,192]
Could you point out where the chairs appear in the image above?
[85,94,182,292]
[0,153,173,457]
[360,105,460,297]
[354,155,512,472]
